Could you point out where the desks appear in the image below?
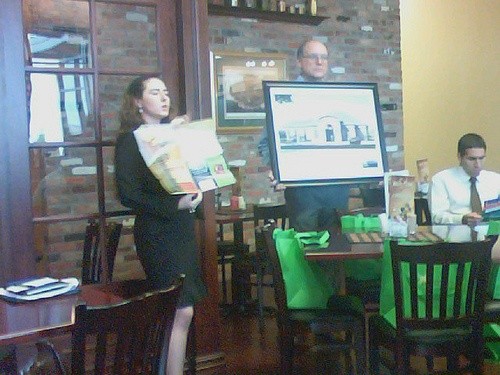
[0,280,172,375]
[304,226,500,296]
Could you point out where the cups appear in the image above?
[381,209,417,238]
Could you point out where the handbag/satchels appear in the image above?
[486,220,500,300]
[482,322,500,366]
[270,226,335,312]
[340,212,383,280]
[378,236,476,330]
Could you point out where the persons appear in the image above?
[428,133,500,227]
[256,39,393,340]
[115,71,203,375]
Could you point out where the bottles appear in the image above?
[208,0,317,16]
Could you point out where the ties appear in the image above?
[470,177,483,214]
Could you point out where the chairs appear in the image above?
[368,236,493,375]
[236,204,286,334]
[259,218,366,375]
[81,216,123,281]
[70,272,187,375]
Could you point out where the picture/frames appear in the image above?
[262,80,389,189]
[208,49,289,135]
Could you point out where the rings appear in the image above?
[189,208,196,214]
